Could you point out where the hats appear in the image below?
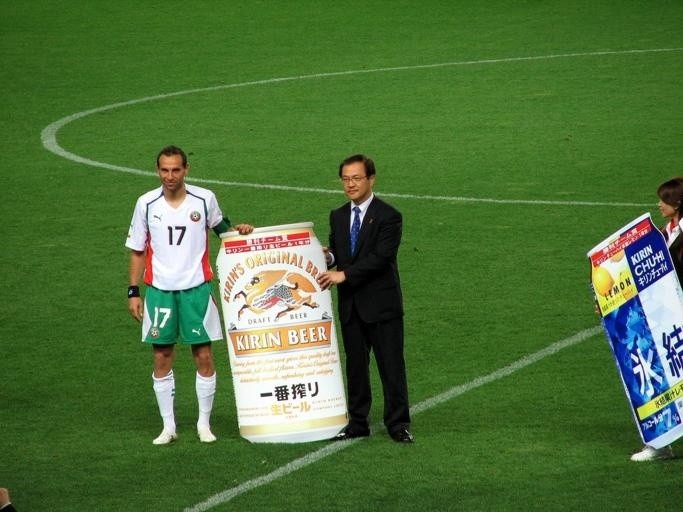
[350,206,361,256]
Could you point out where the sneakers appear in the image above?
[630,444,675,462]
[197,422,217,442]
[153,427,177,445]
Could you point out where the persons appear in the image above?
[1,486,16,512]
[317,153,416,444]
[628,176,683,464]
[125,146,256,446]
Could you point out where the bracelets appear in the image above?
[126,286,140,299]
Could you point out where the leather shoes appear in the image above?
[330,429,370,441]
[389,428,415,443]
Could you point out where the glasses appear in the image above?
[340,176,367,183]
